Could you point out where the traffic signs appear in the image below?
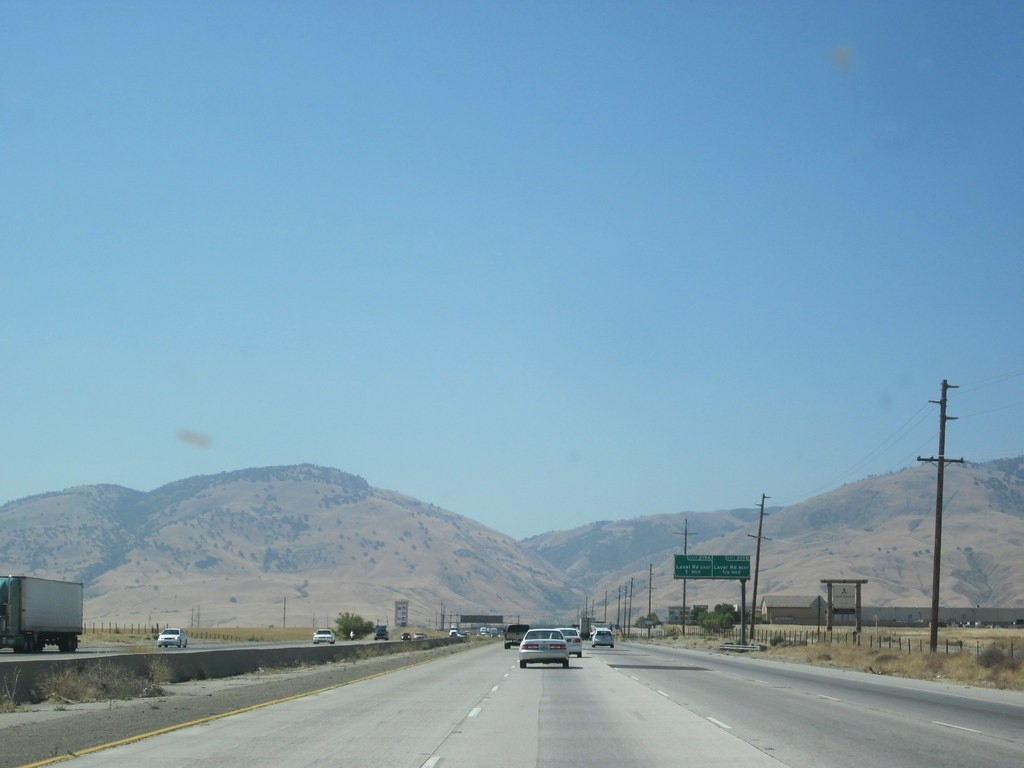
[673,551,753,581]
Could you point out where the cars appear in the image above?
[374,629,390,641]
[402,633,411,641]
[447,626,506,639]
[414,632,425,640]
[157,628,188,648]
[553,627,583,657]
[592,627,615,648]
[518,629,570,669]
[313,628,335,645]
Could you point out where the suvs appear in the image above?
[503,624,530,650]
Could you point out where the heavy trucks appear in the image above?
[0,575,85,655]
